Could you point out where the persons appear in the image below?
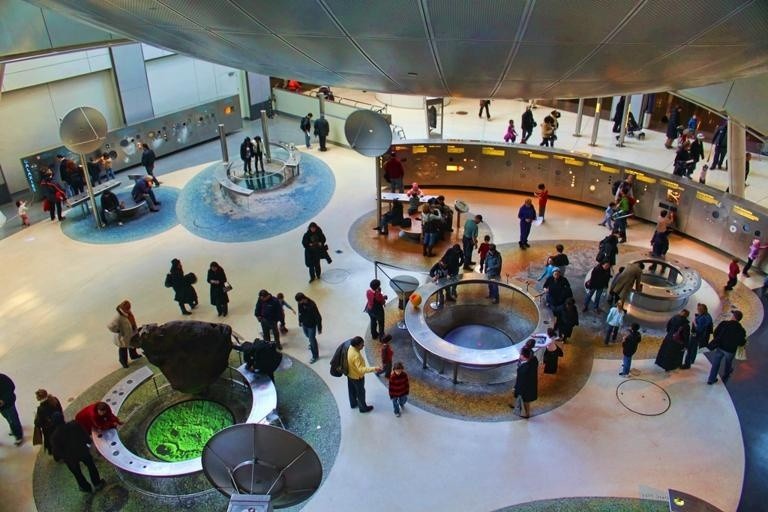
[609,94,749,184]
[164,259,201,315]
[107,299,142,369]
[132,175,162,212]
[1,371,25,445]
[238,278,412,422]
[301,221,333,283]
[478,100,490,119]
[15,199,30,226]
[725,232,767,289]
[240,137,255,175]
[46,410,108,492]
[77,399,121,434]
[300,113,313,148]
[380,153,503,304]
[504,105,561,149]
[655,304,746,385]
[251,136,265,171]
[140,144,159,188]
[519,174,673,375]
[37,141,123,228]
[513,347,539,418]
[314,115,330,151]
[206,261,232,317]
[33,389,66,455]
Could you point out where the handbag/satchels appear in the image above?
[671,326,686,344]
[585,279,591,288]
[43,199,50,211]
[164,273,171,287]
[56,191,66,202]
[400,218,411,227]
[222,286,233,293]
[33,425,42,445]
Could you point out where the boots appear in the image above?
[423,243,436,257]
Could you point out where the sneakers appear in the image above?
[360,406,373,412]
[379,232,388,235]
[309,357,317,364]
[309,276,320,283]
[154,201,161,205]
[486,294,497,304]
[582,304,588,312]
[373,227,382,230]
[277,343,283,350]
[95,478,105,490]
[446,290,457,301]
[14,437,23,445]
[182,303,197,314]
[101,223,106,227]
[519,240,530,249]
[218,312,227,316]
[464,262,475,271]
[679,364,691,369]
[150,209,160,212]
[554,336,566,343]
[620,239,626,243]
[592,307,599,314]
[279,326,288,334]
[395,403,405,416]
[119,354,142,368]
[117,222,124,225]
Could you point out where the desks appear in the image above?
[65,178,121,216]
[380,191,440,211]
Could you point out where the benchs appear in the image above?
[399,210,423,239]
[105,190,150,222]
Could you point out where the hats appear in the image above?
[476,215,483,222]
[144,175,153,182]
